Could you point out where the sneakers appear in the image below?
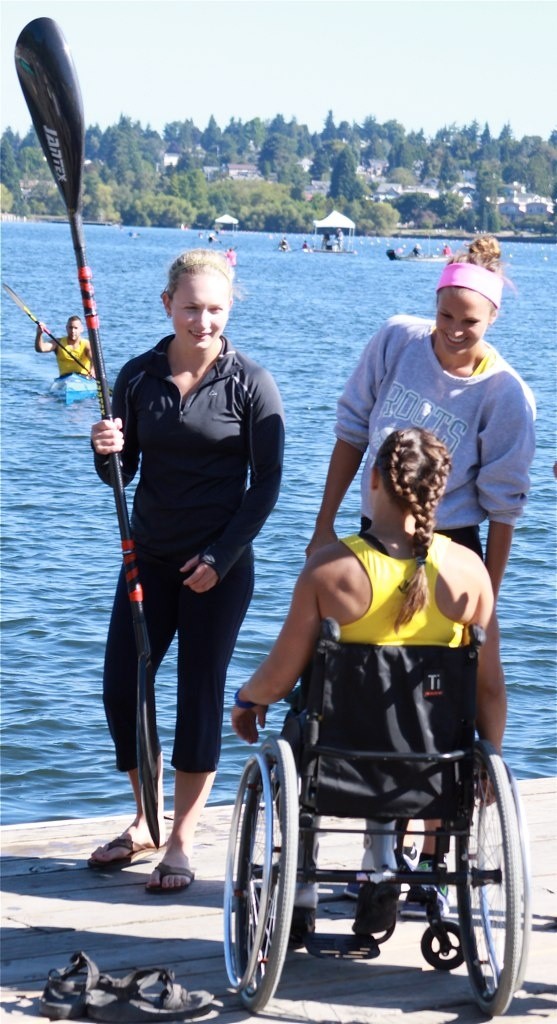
[398,860,450,917]
[344,882,365,898]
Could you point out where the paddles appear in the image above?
[11,15,162,847]
[2,284,113,396]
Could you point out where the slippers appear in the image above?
[145,861,196,894]
[87,838,168,871]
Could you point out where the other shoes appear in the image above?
[288,904,317,948]
[351,878,401,935]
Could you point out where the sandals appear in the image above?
[86,967,212,1024]
[38,949,140,1020]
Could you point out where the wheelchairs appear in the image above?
[222,616,533,1017]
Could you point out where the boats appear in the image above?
[49,371,99,406]
[385,233,449,263]
[311,210,359,254]
[214,214,239,237]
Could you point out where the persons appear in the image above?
[304,238,537,920]
[303,240,308,248]
[413,248,420,257]
[443,245,450,257]
[232,429,507,934]
[35,316,96,378]
[226,249,235,259]
[324,229,345,251]
[88,249,285,893]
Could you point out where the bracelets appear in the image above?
[234,688,256,709]
